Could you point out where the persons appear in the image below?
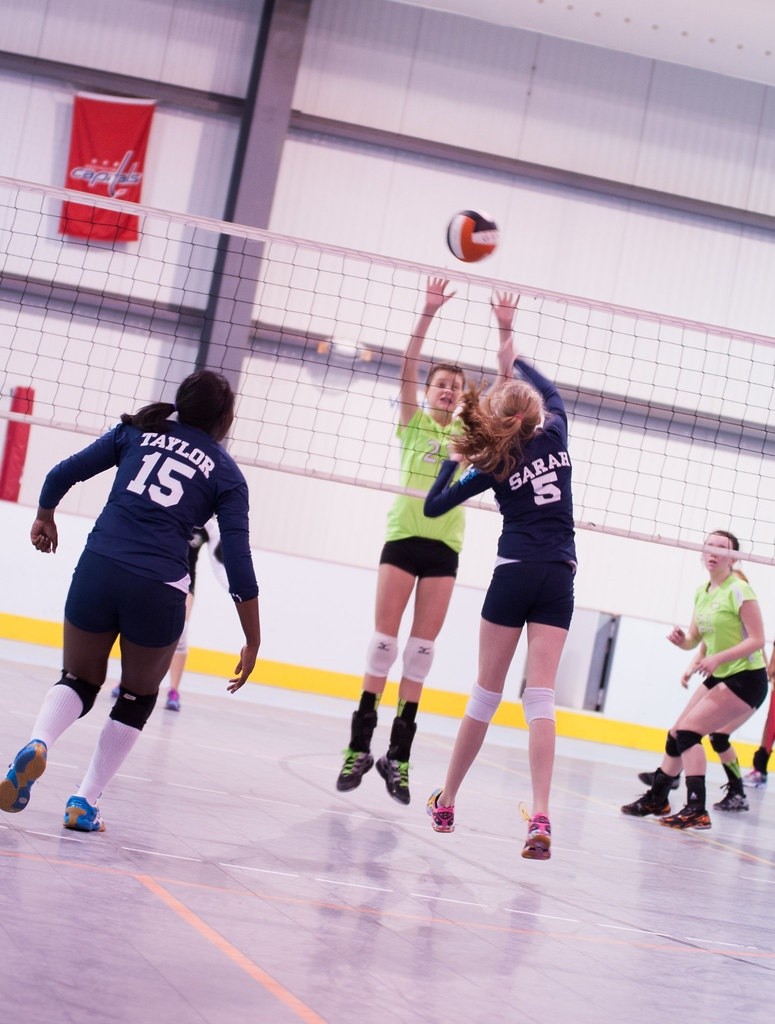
[620,531,775,830]
[337,277,578,859]
[0,370,260,832]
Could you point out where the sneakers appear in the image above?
[712,782,749,813]
[638,773,680,790]
[658,804,712,830]
[621,790,672,817]
[60,795,107,832]
[742,770,768,789]
[0,738,48,813]
[375,755,411,805]
[518,802,556,858]
[337,748,375,792]
[166,689,181,711]
[426,788,455,833]
[111,686,119,698]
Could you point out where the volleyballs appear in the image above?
[445,208,503,264]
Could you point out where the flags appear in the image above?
[57,90,158,244]
